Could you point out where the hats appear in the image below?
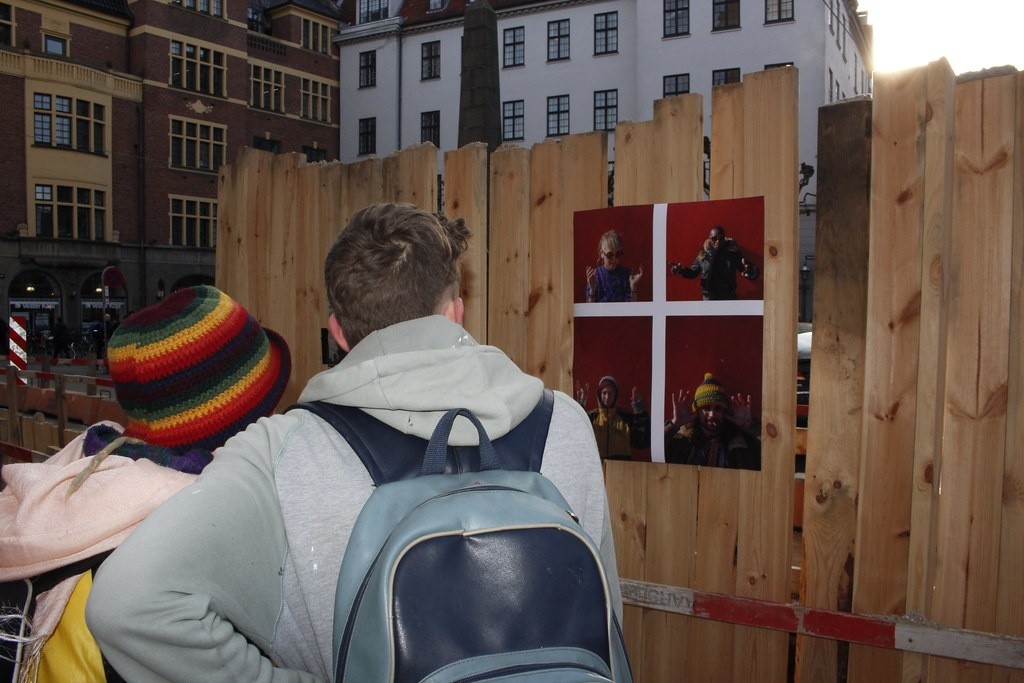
[692,373,732,412]
[598,379,618,394]
[107,286,292,457]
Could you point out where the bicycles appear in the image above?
[28,329,96,359]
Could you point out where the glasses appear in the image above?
[710,236,725,242]
[602,250,622,259]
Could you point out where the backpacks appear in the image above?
[283,389,634,683]
[0,549,116,683]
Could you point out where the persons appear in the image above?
[585,229,647,303]
[664,373,761,472]
[575,375,651,462]
[670,226,761,300]
[83,201,637,682]
[93,313,117,359]
[49,316,71,360]
[1,283,294,682]
